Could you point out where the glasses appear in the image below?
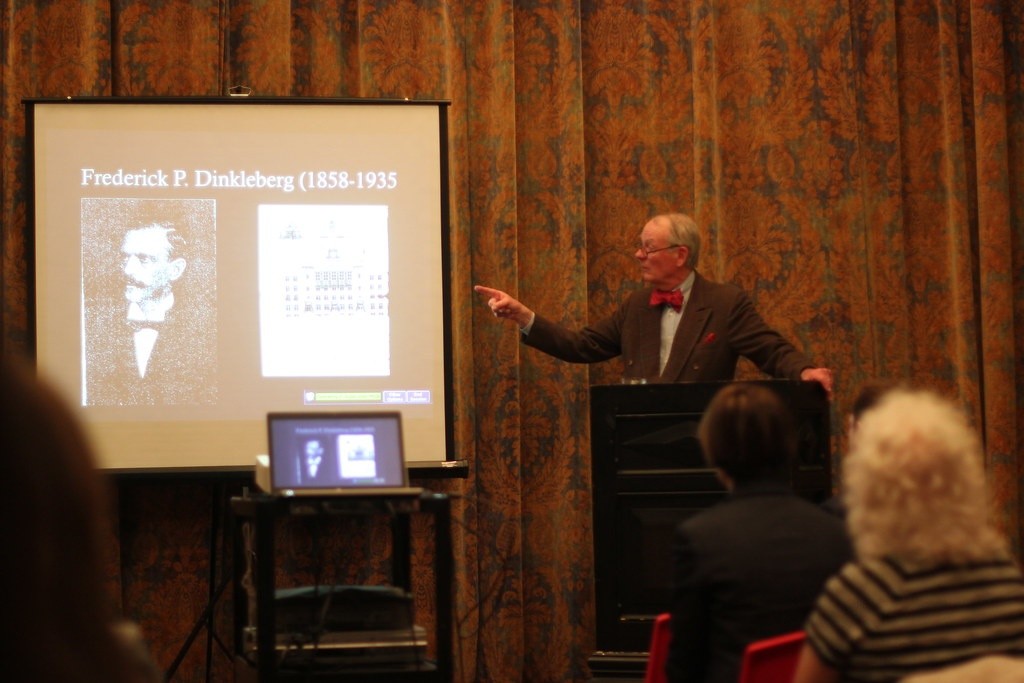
[634,242,680,257]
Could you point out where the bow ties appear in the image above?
[649,287,684,313]
[126,319,165,333]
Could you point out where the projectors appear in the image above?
[254,455,272,494]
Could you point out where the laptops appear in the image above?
[267,411,423,499]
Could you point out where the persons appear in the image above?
[797,392,1024,683]
[86,224,214,403]
[475,214,835,403]
[303,438,328,480]
[0,364,161,683]
[665,382,854,683]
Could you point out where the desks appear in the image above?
[222,491,453,683]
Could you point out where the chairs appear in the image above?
[643,614,807,683]
